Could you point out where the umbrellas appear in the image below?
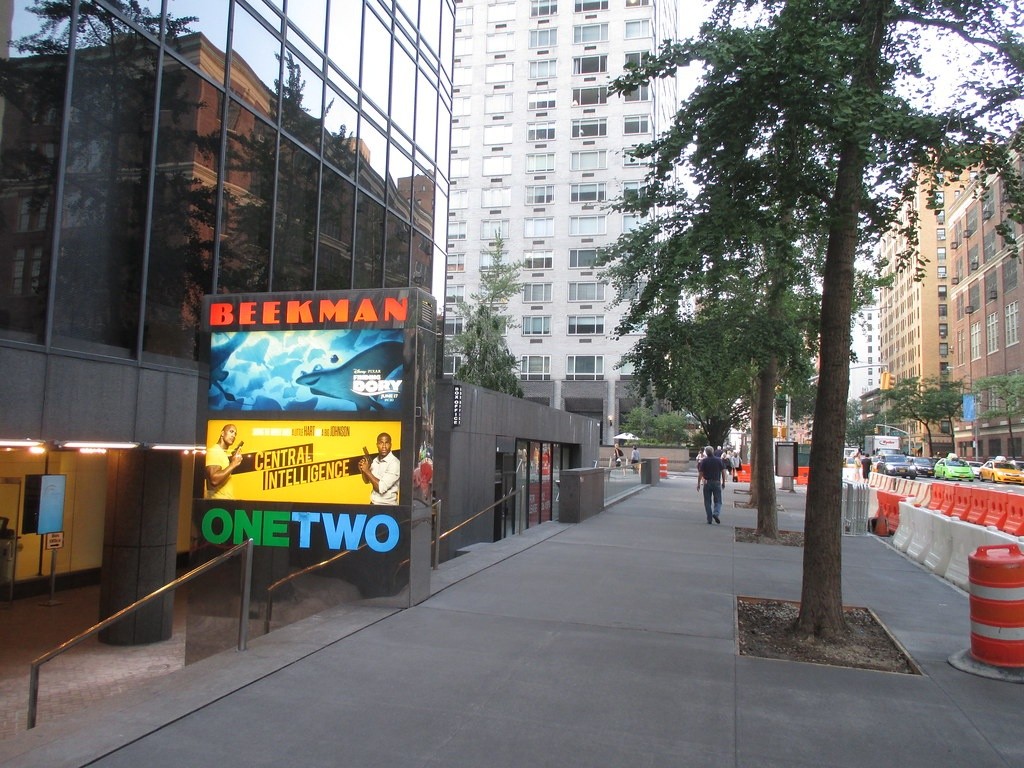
[613,432,641,445]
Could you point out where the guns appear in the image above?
[231,441,244,456]
[362,446,373,465]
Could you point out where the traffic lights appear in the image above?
[874,426,879,434]
[879,372,896,391]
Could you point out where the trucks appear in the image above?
[865,435,903,460]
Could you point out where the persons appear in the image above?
[697,446,726,524]
[517,446,539,474]
[630,444,640,474]
[861,454,873,483]
[696,445,742,482]
[357,433,400,505]
[613,443,622,469]
[206,423,243,500]
[854,453,861,481]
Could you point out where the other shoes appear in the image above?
[713,514,721,523]
[705,520,712,525]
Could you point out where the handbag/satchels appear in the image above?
[736,464,742,471]
[721,453,729,463]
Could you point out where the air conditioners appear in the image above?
[965,306,974,314]
[941,274,948,278]
[890,335,893,339]
[256,134,265,142]
[896,347,899,352]
[901,361,903,365]
[950,278,958,285]
[970,262,978,270]
[988,291,996,300]
[963,230,970,238]
[982,211,992,220]
[950,242,957,249]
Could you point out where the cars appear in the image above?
[978,456,1024,484]
[879,454,917,481]
[932,457,941,464]
[933,452,974,482]
[909,456,935,478]
[844,448,879,471]
[965,461,984,478]
